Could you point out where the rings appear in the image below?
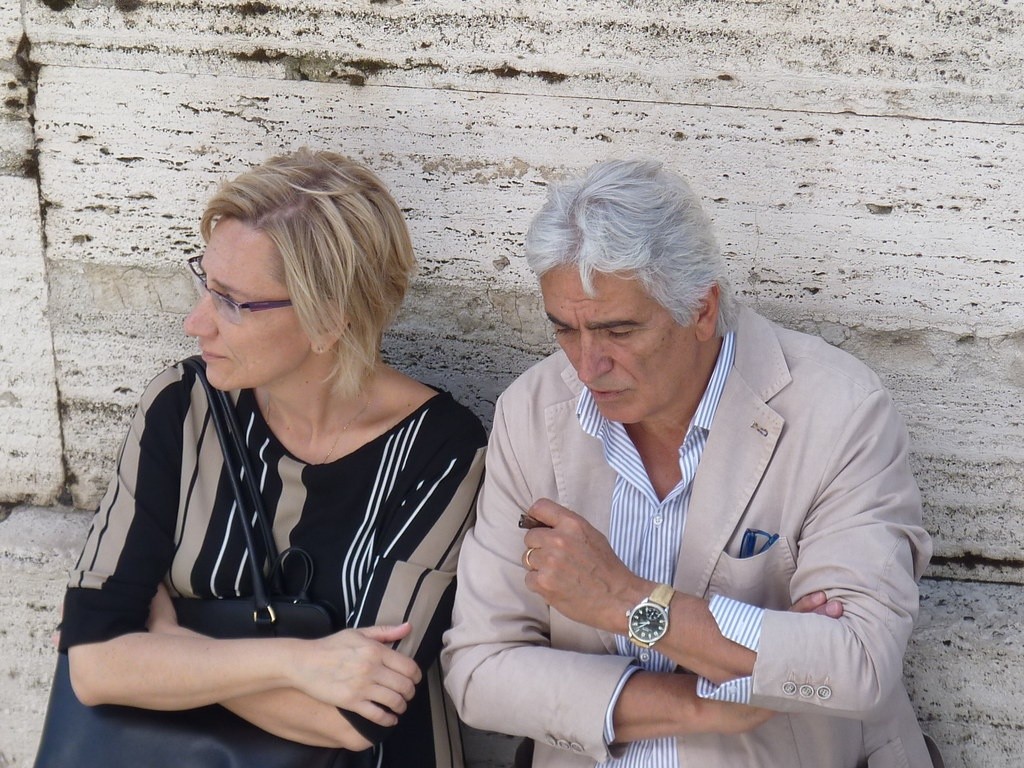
[526,548,537,571]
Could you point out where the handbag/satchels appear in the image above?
[34,350,335,768]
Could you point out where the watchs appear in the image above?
[626,583,675,647]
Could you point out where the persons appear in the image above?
[440,160,934,768]
[53,151,489,768]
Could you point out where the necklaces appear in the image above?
[265,394,372,464]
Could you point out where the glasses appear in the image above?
[188,254,293,328]
[737,527,779,558]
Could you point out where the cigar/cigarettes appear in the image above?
[519,513,547,529]
